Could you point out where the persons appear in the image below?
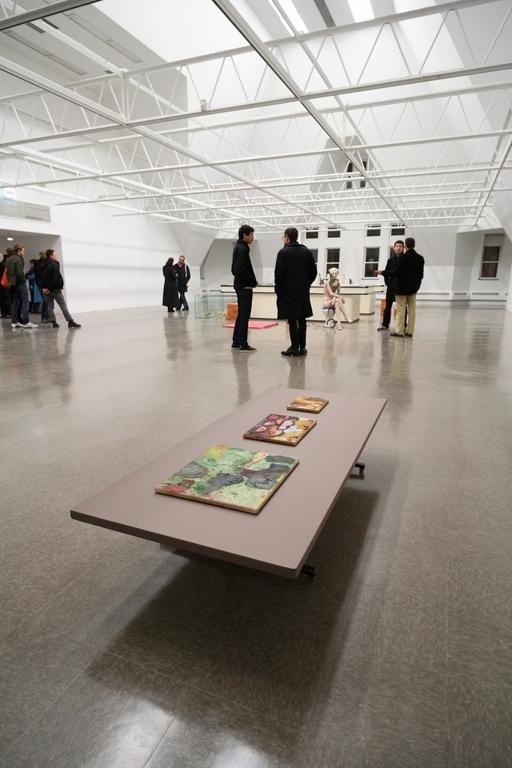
[321,267,353,330]
[231,224,257,351]
[1,243,48,329]
[37,249,82,328]
[374,237,424,337]
[377,240,408,333]
[274,227,318,357]
[161,257,180,312]
[174,255,190,311]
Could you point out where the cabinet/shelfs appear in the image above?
[221,283,375,323]
[359,284,384,315]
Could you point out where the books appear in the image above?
[242,413,317,447]
[287,394,329,413]
[154,445,300,515]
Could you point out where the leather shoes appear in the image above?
[68,322,81,327]
[377,327,412,337]
[167,307,189,312]
[281,348,308,357]
[231,344,256,350]
[53,323,60,328]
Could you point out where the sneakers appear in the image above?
[11,323,38,328]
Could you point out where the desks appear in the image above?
[70,385,388,578]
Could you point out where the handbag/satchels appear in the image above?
[1,268,9,288]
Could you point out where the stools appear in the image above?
[322,306,337,328]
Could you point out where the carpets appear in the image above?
[224,319,279,328]
